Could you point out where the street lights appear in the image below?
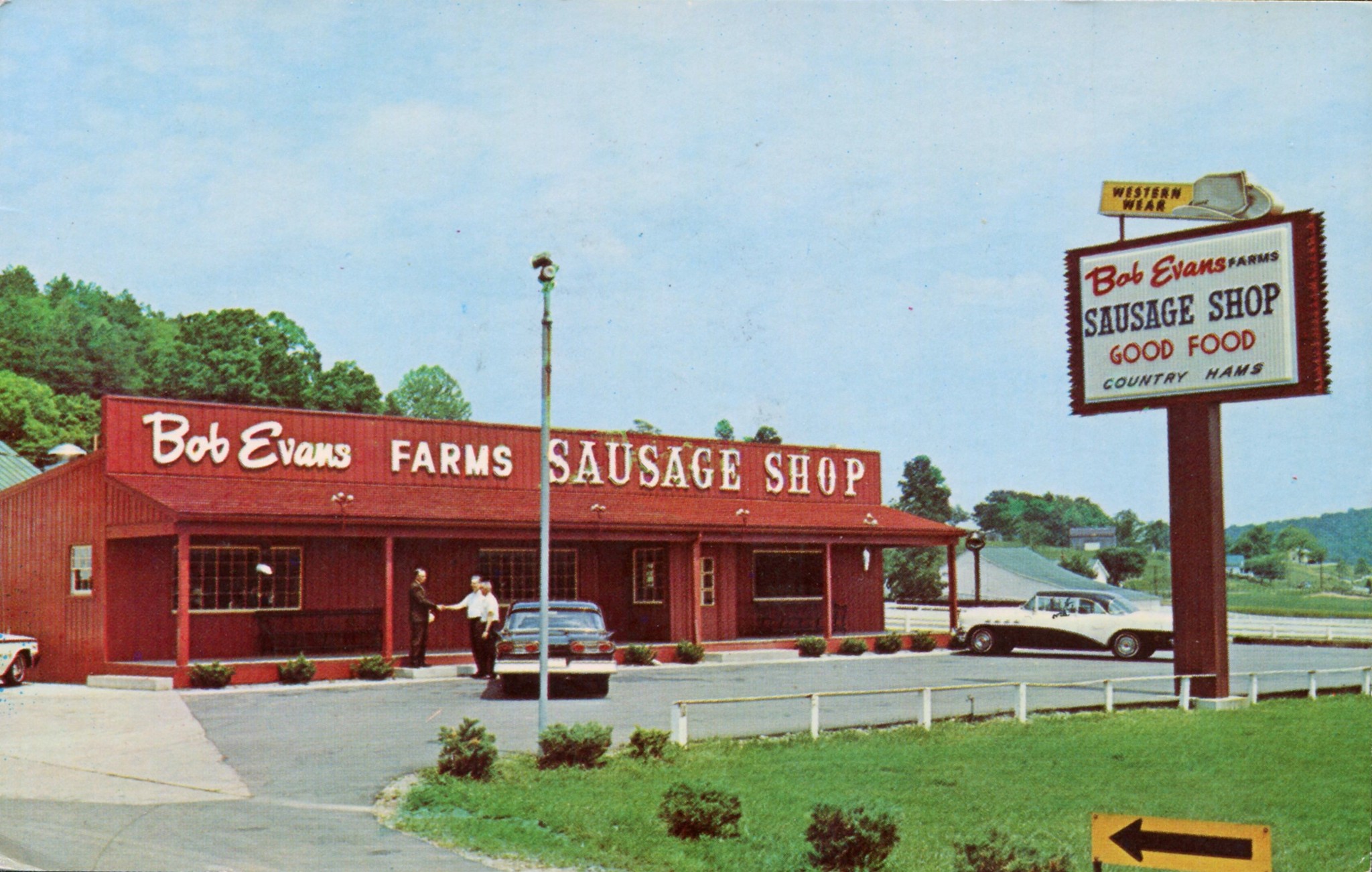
[965,530,987,600]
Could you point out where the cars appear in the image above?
[492,598,619,697]
[956,588,1173,661]
[0,632,42,686]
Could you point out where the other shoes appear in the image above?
[421,662,431,667]
[481,674,492,679]
[412,663,420,668]
[470,674,483,680]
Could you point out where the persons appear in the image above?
[445,573,500,678]
[409,568,445,668]
